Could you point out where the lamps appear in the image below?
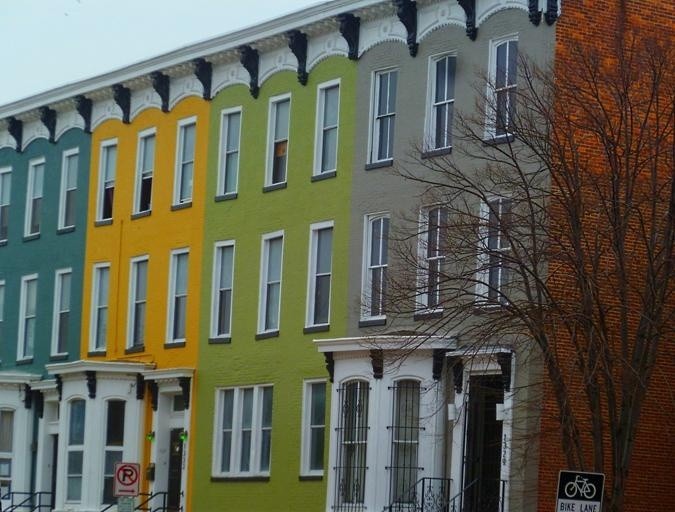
[447,403,457,421]
[142,431,156,442]
[178,431,188,441]
[495,401,508,421]
